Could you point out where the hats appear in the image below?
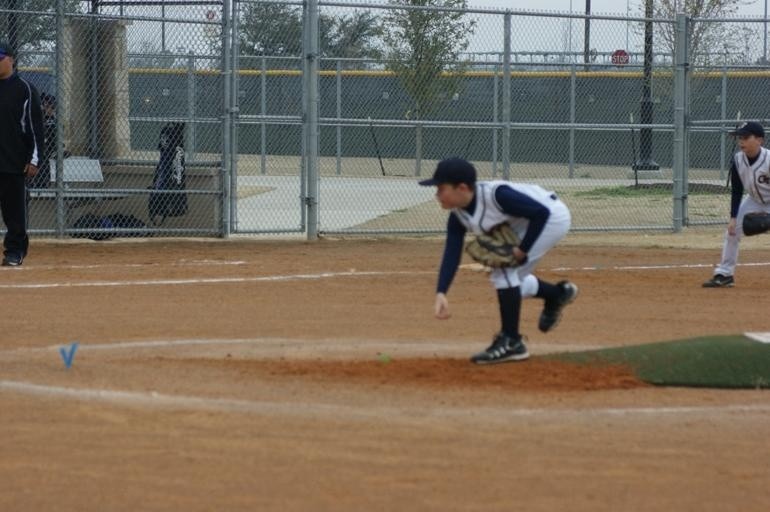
[0,39,13,58]
[729,122,764,139]
[416,159,477,186]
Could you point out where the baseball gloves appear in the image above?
[743,212,770,235]
[464,222,528,269]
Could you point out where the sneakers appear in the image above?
[701,273,738,288]
[0,250,26,267]
[535,280,579,333]
[469,332,532,364]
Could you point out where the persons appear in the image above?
[415,155,580,365]
[700,117,770,289]
[0,41,48,269]
[29,89,56,188]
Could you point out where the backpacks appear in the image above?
[147,120,188,226]
